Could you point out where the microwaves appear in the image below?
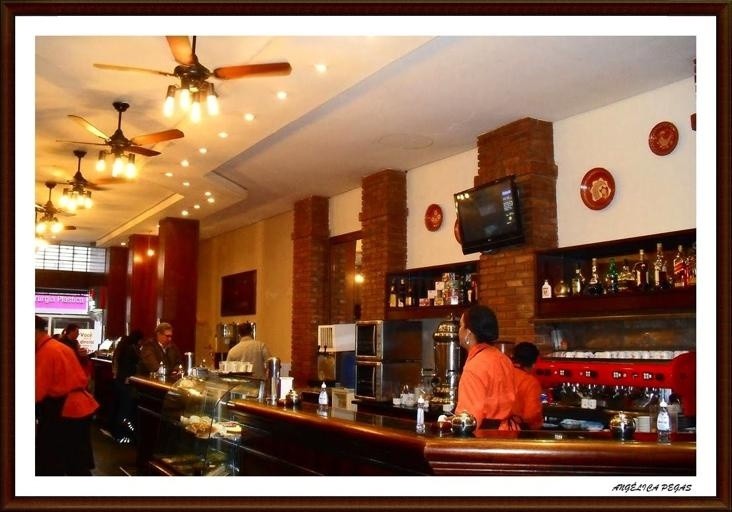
[353,319,423,360]
[354,362,421,399]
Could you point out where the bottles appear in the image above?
[656,400,671,442]
[390,280,396,307]
[406,275,414,309]
[542,280,552,298]
[318,382,330,414]
[286,390,299,406]
[396,278,405,308]
[572,244,696,296]
[164,365,166,375]
[458,276,464,304]
[176,364,185,378]
[159,361,163,376]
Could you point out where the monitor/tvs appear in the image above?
[453,174,526,254]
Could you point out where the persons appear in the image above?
[36,315,102,475]
[454,304,518,430]
[57,323,88,369]
[137,322,184,382]
[509,341,545,430]
[226,323,272,385]
[111,330,145,448]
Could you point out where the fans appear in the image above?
[55,102,184,156]
[35,150,128,191]
[35,181,76,216]
[93,36,292,101]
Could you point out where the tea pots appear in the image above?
[554,280,572,298]
[405,398,417,407]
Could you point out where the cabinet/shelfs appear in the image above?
[535,228,696,429]
[383,260,478,437]
[148,375,259,476]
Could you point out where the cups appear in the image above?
[443,405,453,412]
[393,398,402,404]
[400,394,408,404]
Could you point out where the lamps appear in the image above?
[58,188,93,211]
[95,151,137,179]
[35,212,63,236]
[163,78,219,121]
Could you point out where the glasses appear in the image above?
[164,334,171,337]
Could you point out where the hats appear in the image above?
[514,342,539,365]
[464,304,498,341]
[35,316,48,328]
